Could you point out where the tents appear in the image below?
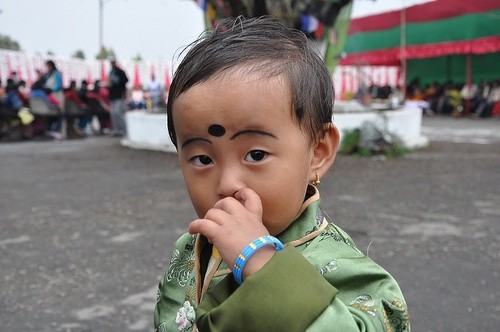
[333,0,500,117]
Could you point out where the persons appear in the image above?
[335,76,500,119]
[42,60,68,140]
[0,68,112,141]
[106,58,129,137]
[151,15,412,332]
[124,74,170,111]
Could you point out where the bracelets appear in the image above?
[231,234,284,286]
